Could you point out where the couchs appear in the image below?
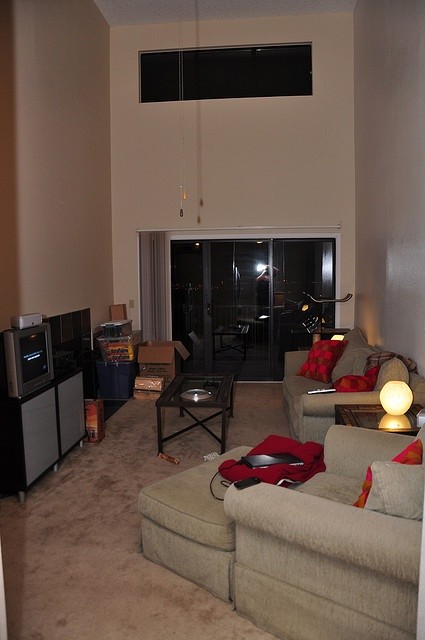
[138,445,298,606]
[283,325,424,446]
[222,422,425,639]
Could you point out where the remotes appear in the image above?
[306,387,337,396]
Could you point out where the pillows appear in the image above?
[370,461,423,520]
[299,340,350,383]
[355,439,423,508]
[332,342,376,381]
[342,327,371,348]
[378,357,410,389]
[329,365,380,392]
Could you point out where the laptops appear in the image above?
[239,450,304,470]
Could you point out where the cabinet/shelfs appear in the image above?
[333,403,424,435]
[1,368,88,503]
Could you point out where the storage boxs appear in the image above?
[95,329,142,362]
[137,339,189,387]
[100,318,134,338]
[97,358,139,400]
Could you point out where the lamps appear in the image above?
[379,413,412,429]
[253,262,269,273]
[379,378,415,416]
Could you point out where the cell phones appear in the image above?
[234,475,260,490]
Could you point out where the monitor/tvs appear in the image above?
[1,323,56,399]
